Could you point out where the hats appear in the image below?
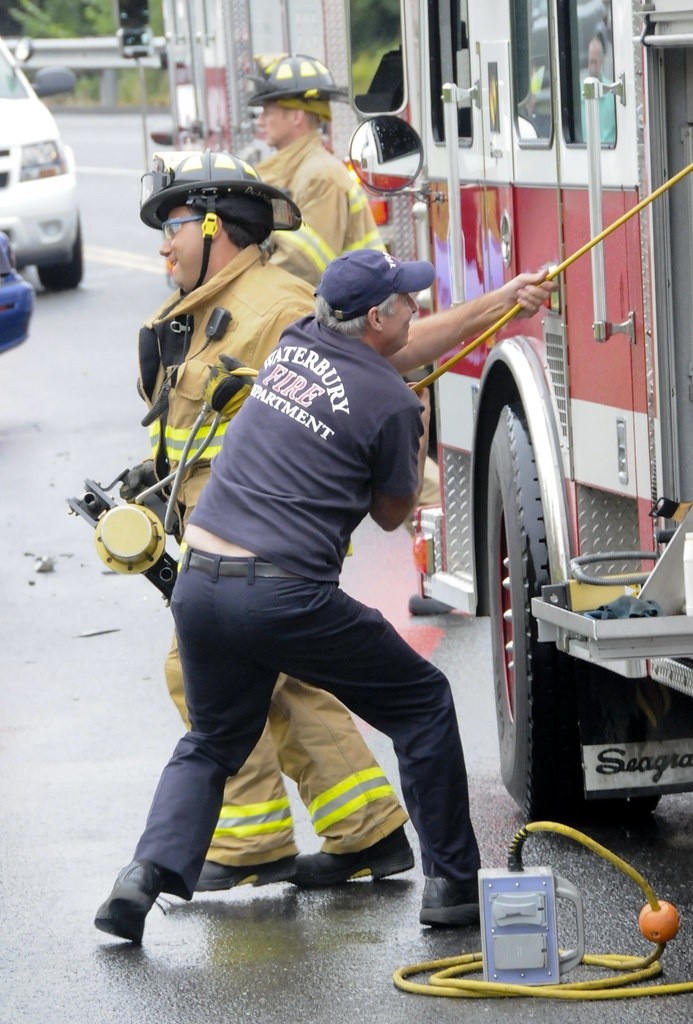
[312,250,436,322]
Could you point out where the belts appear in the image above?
[182,551,304,579]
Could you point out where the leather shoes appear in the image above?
[419,875,479,927]
[92,859,162,942]
[283,827,416,890]
[188,857,297,890]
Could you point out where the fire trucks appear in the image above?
[350,0,693,827]
[159,0,425,314]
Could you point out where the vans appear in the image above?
[1,37,94,292]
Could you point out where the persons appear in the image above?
[89,244,558,947]
[248,51,395,292]
[109,151,416,894]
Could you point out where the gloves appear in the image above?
[119,460,172,509]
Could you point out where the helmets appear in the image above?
[140,153,301,239]
[247,52,349,106]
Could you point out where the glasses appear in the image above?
[160,214,206,241]
